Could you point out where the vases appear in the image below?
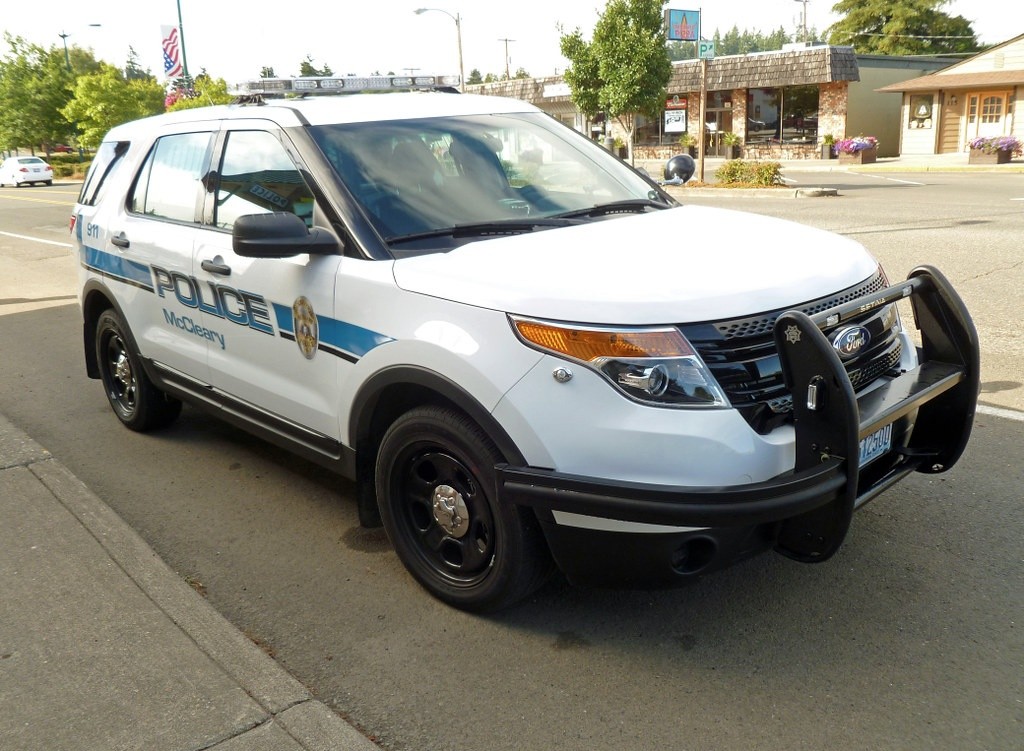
[839,147,877,164]
[970,147,1012,164]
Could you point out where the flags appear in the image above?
[161,24,182,78]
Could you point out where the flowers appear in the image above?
[830,136,880,155]
[964,136,1024,158]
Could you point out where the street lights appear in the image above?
[413,7,467,95]
[57,23,102,75]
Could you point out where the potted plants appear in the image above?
[822,134,837,159]
[722,132,740,159]
[613,137,625,159]
[678,133,697,158]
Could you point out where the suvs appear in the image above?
[69,73,982,614]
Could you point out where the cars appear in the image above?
[79,146,95,153]
[0,156,54,188]
[49,143,75,154]
